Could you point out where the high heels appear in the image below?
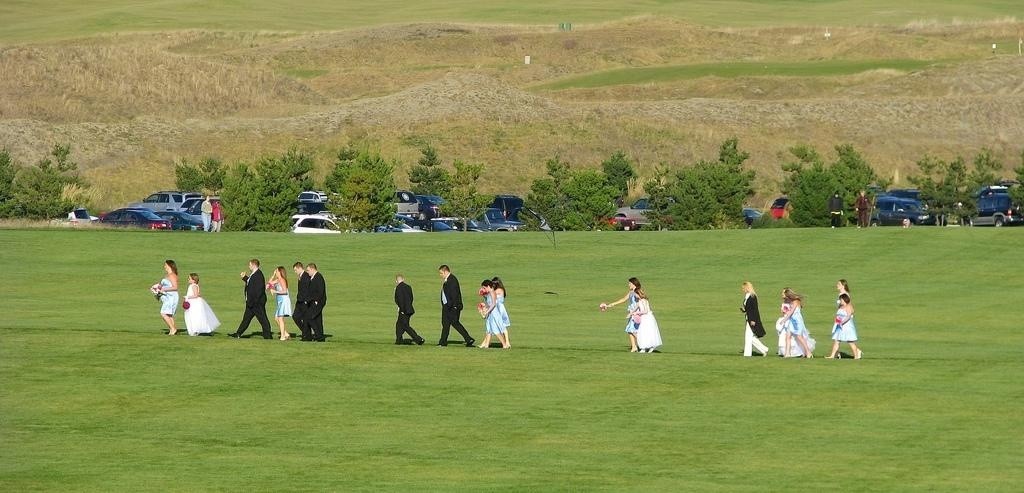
[280,337,286,340]
[836,354,841,359]
[478,344,486,348]
[286,334,291,339]
[171,329,177,335]
[507,345,511,348]
[783,354,791,357]
[857,349,864,360]
[168,330,171,335]
[630,346,638,352]
[805,353,814,358]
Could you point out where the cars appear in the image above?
[180,198,223,225]
[154,210,204,231]
[99,208,172,230]
[396,191,423,214]
[291,214,367,233]
[614,196,675,224]
[607,216,636,231]
[468,208,524,231]
[871,195,935,225]
[299,191,342,212]
[965,185,1023,227]
[998,179,1020,188]
[64,208,99,222]
[486,195,525,208]
[417,194,448,215]
[742,208,762,224]
[430,217,490,232]
[374,215,429,232]
[887,189,923,199]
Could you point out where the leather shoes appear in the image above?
[762,347,769,356]
[639,349,645,352]
[227,333,240,338]
[418,338,425,345]
[437,343,447,346]
[648,345,657,354]
[465,338,474,347]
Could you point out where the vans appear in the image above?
[769,198,788,218]
[128,191,203,211]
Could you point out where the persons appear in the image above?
[201,195,213,232]
[825,294,858,360]
[211,200,224,232]
[834,280,865,359]
[481,279,507,350]
[775,298,816,358]
[740,281,770,358]
[182,273,221,337]
[436,265,475,347]
[160,260,179,336]
[292,262,311,342]
[394,275,425,346]
[782,287,814,359]
[856,192,876,228]
[606,278,641,353]
[829,192,843,229]
[226,259,273,339]
[479,276,511,350]
[268,266,292,340]
[306,263,327,342]
[634,289,663,353]
